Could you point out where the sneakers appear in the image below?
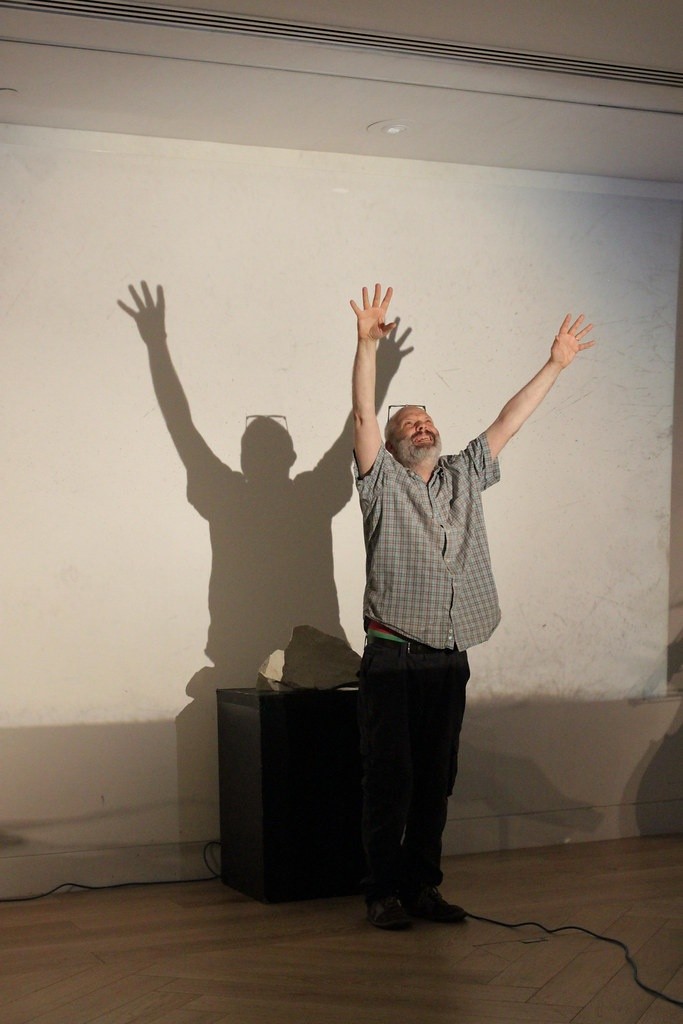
[367,897,409,928]
[397,883,465,921]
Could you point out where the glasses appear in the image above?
[388,404,426,423]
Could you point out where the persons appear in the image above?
[348,283,598,930]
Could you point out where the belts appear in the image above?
[366,636,434,653]
